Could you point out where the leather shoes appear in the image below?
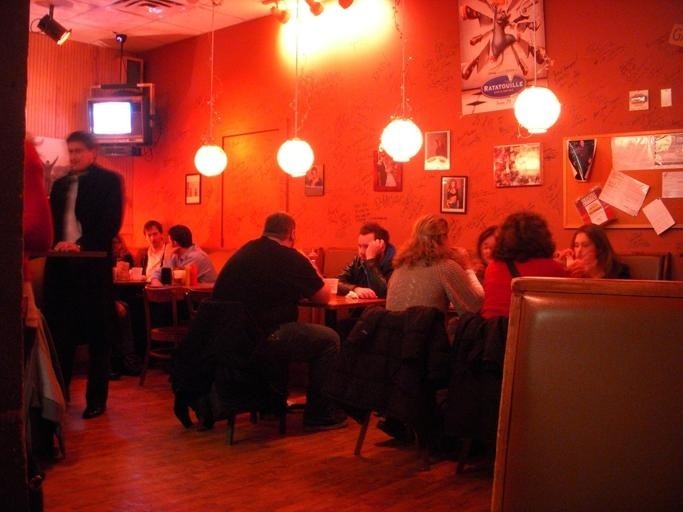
[83,408,104,417]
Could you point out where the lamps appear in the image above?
[510,2,563,136]
[36,4,72,45]
[191,0,232,181]
[374,1,427,165]
[273,2,319,179]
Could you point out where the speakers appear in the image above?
[117,57,145,91]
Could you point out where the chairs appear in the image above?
[356,306,439,471]
[615,249,672,280]
[188,299,286,445]
[446,313,508,484]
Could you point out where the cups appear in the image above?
[322,278,338,293]
[112,259,186,287]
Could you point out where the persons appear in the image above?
[445,180,460,208]
[382,155,399,188]
[307,166,322,187]
[333,220,396,300]
[140,219,168,282]
[480,210,573,322]
[377,212,485,443]
[148,224,216,289]
[554,223,632,280]
[38,128,123,421]
[209,211,348,433]
[110,234,136,380]
[19,133,55,295]
[476,222,497,264]
[573,141,587,175]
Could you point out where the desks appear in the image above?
[138,283,215,386]
[113,278,155,336]
[298,295,385,331]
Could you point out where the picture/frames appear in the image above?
[490,141,545,179]
[184,174,202,205]
[440,175,468,215]
[421,128,453,171]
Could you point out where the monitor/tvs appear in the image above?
[87,94,152,144]
